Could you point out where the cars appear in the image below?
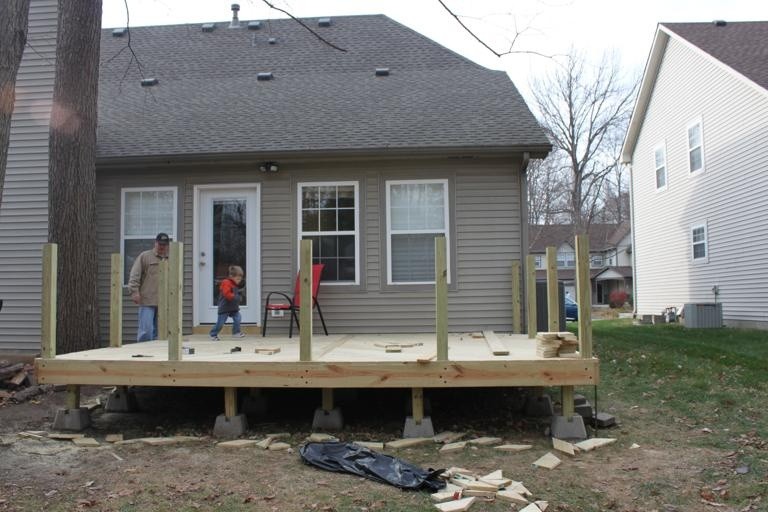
[564,296,578,320]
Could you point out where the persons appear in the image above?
[208,265,247,341]
[127,232,169,342]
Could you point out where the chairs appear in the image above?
[262,264,329,338]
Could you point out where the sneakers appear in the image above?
[232,332,245,337]
[208,333,220,340]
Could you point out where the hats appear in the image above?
[156,233,170,245]
